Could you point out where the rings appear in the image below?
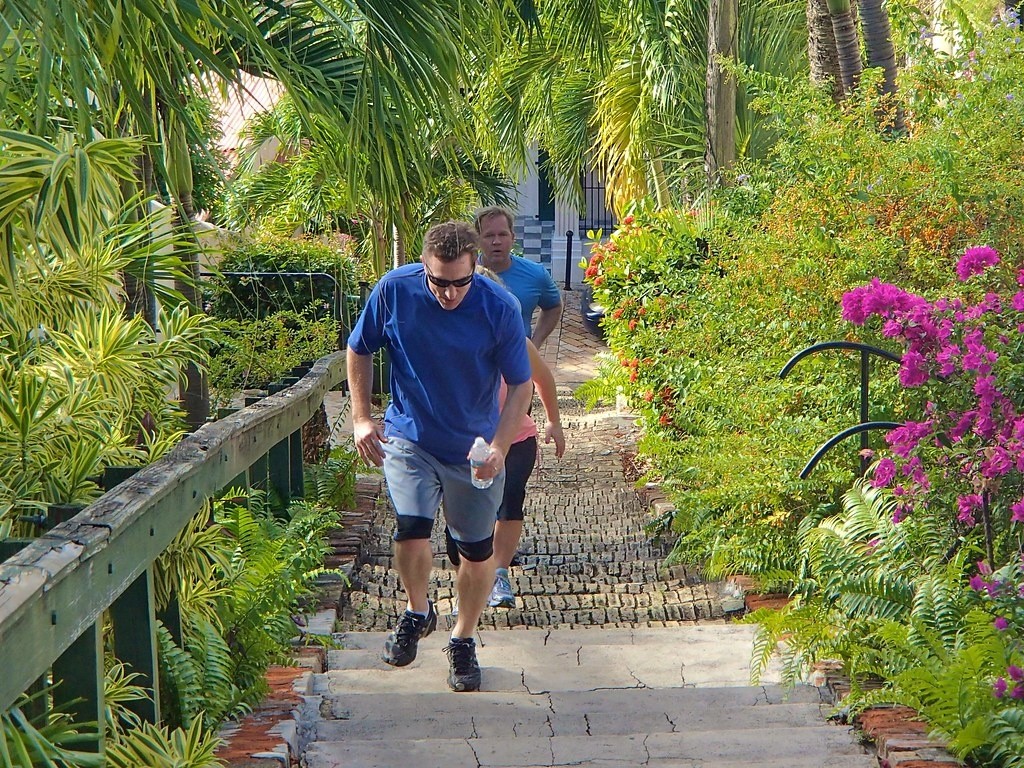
[494,466,498,471]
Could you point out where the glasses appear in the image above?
[426,262,476,288]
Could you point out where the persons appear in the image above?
[473,207,562,418]
[346,221,534,692]
[446,266,565,614]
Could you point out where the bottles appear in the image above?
[470,436,493,489]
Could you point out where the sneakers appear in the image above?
[451,605,458,615]
[488,572,516,609]
[442,632,481,692]
[381,600,437,667]
